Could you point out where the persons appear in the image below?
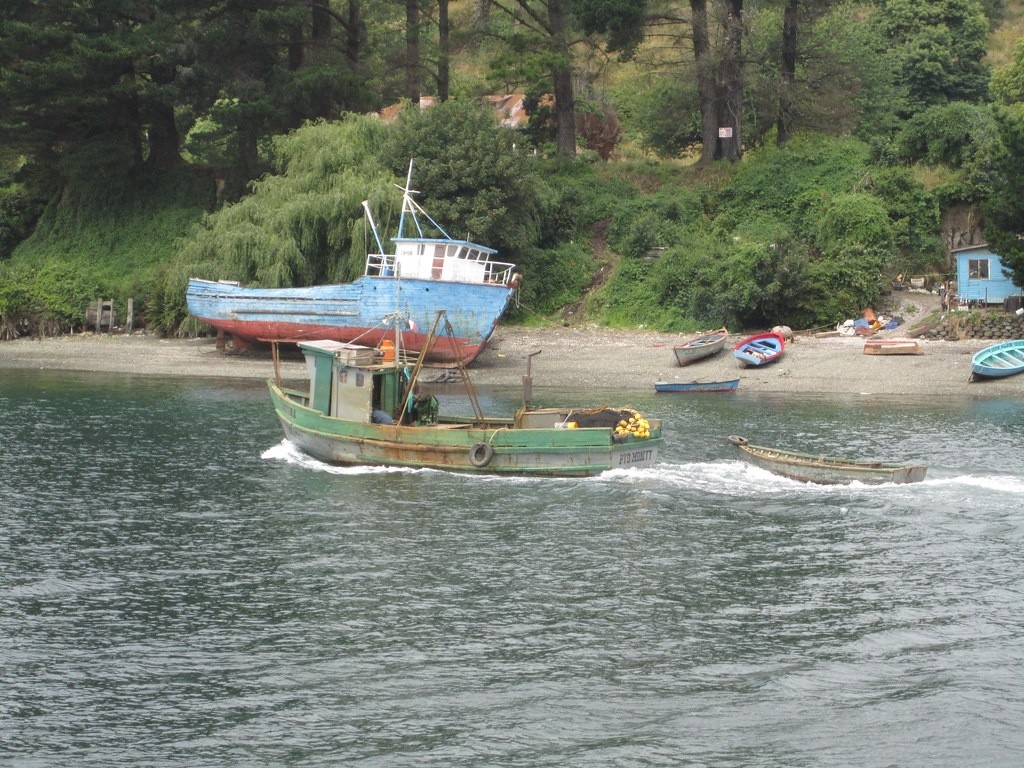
[372,409,393,425]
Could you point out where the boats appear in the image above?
[267,262,663,478]
[673,326,728,367]
[863,341,924,355]
[728,435,928,485]
[734,332,785,366]
[654,379,741,392]
[968,340,1024,381]
[186,158,522,369]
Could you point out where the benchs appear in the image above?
[692,339,714,346]
[855,462,881,467]
[982,349,1024,367]
[749,341,776,355]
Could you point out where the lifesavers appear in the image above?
[469,442,493,468]
